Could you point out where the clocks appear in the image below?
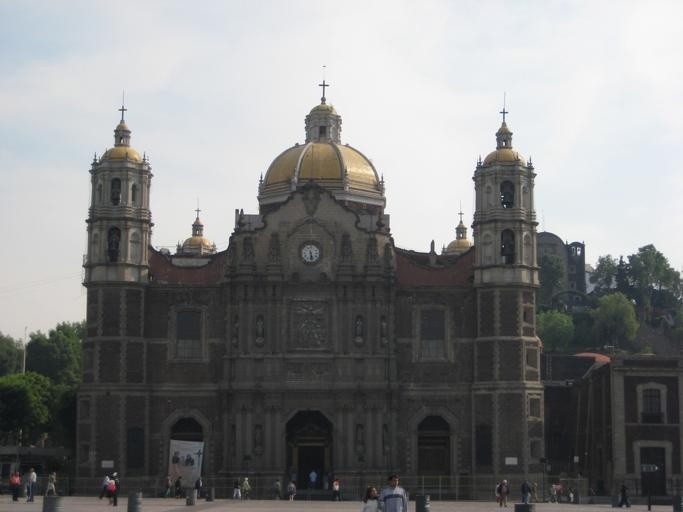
[297,240,324,266]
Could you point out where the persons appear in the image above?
[233,475,242,501]
[44,470,57,497]
[174,475,183,498]
[195,476,203,498]
[10,470,22,502]
[272,476,283,501]
[26,466,37,503]
[286,479,296,500]
[172,451,194,466]
[360,486,380,511]
[240,477,251,500]
[309,468,318,489]
[617,484,632,508]
[377,474,409,512]
[494,479,579,507]
[99,472,120,507]
[331,477,341,501]
